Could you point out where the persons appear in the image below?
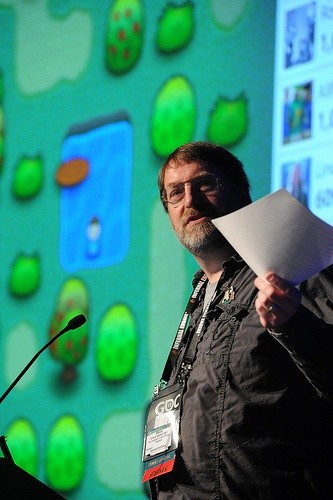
[142,142,333,500]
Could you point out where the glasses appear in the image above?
[160,174,237,203]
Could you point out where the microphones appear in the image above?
[0,314,87,404]
